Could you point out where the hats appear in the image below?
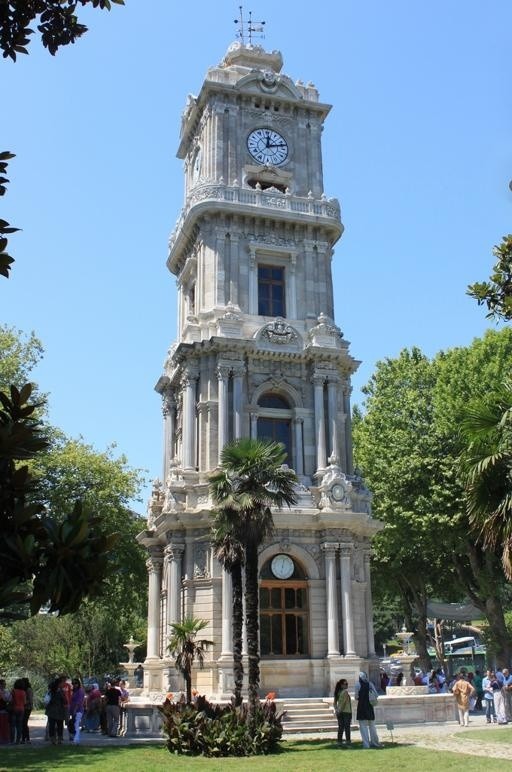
[358,672,368,681]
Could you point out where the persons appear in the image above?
[134,665,144,688]
[382,668,512,727]
[355,672,380,749]
[333,679,352,746]
[0,674,129,745]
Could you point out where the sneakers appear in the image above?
[337,741,384,748]
[9,734,79,745]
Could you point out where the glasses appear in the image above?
[342,683,348,686]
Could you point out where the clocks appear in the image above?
[246,126,288,165]
[271,553,294,579]
[191,148,202,184]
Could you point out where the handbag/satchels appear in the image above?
[455,683,460,697]
[5,691,14,712]
[368,688,379,707]
